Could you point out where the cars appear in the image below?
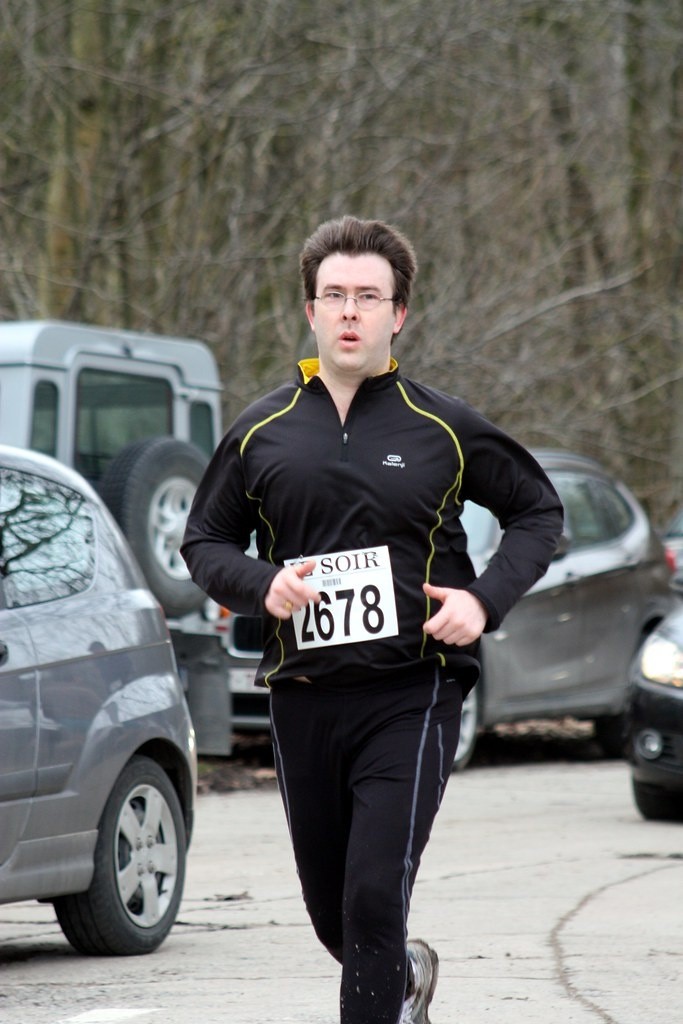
[655,500,683,586]
[228,448,683,773]
[0,444,197,957]
[623,604,683,825]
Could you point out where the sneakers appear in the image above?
[396,939,440,1024]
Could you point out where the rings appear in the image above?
[285,600,292,612]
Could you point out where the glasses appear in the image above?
[313,291,396,311]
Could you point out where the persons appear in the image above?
[179,215,563,1024]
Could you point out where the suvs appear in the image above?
[0,313,237,759]
